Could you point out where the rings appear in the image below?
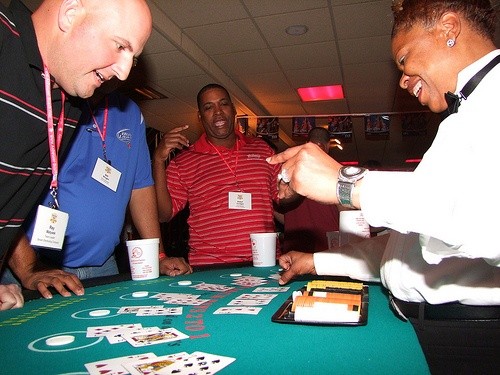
[281,169,290,183]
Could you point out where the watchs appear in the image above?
[336,165,370,210]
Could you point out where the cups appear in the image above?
[250,233,277,267]
[327,231,339,250]
[338,210,371,248]
[126,238,160,280]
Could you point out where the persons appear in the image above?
[152,84,297,268]
[0,54,193,301]
[273,127,340,253]
[0,0,152,309]
[265,1,500,375]
[256,136,284,251]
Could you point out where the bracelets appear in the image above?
[159,253,168,262]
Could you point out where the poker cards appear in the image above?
[83,273,291,375]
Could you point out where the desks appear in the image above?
[0,264,431,375]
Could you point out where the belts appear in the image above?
[389,292,500,321]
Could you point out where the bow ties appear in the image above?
[442,55,500,121]
